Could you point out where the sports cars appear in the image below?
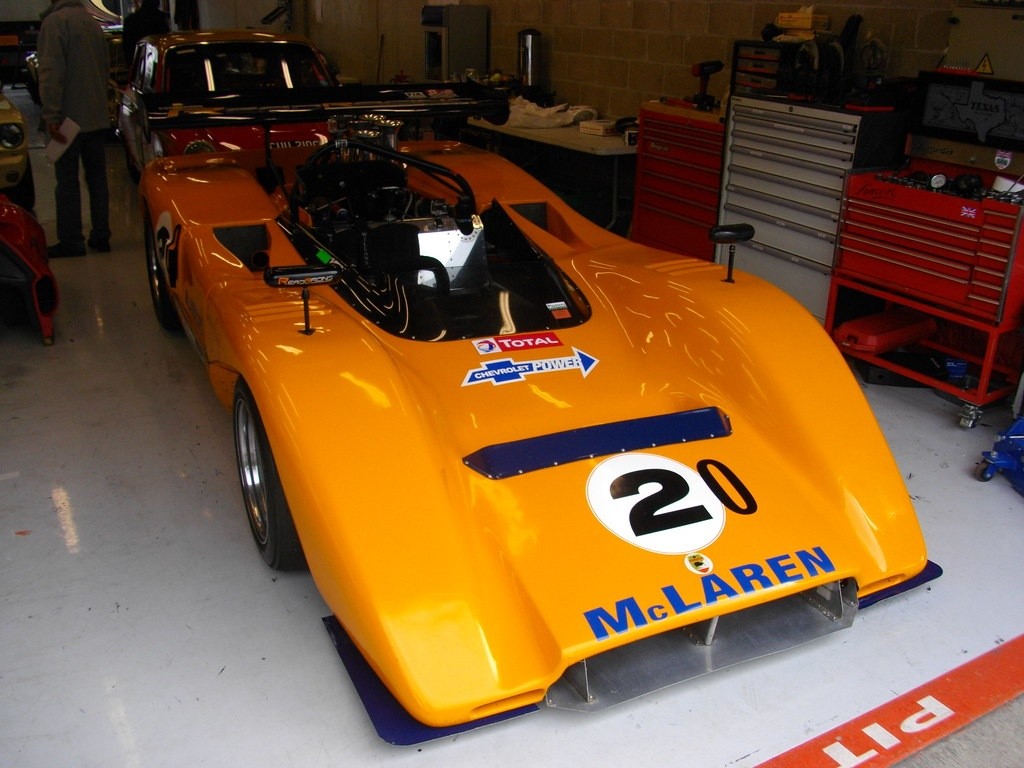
[139,94,946,743]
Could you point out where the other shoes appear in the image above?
[47,239,86,258]
[88,235,111,253]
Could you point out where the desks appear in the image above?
[467,113,638,230]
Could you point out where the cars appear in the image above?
[122,33,364,194]
[0,94,39,212]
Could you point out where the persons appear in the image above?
[34,0,111,258]
[122,0,170,67]
